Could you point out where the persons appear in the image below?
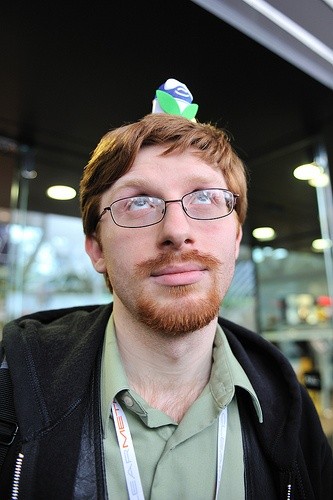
[0,115,333,500]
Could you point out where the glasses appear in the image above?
[94,187,241,229]
